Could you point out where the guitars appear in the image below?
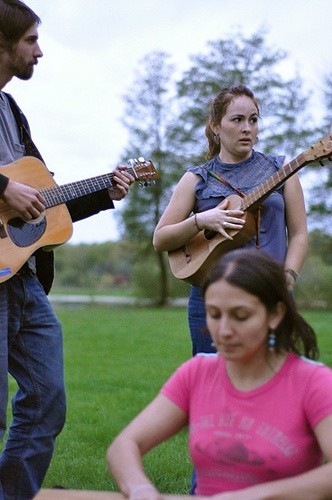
[168,132,332,289]
[0,156,158,283]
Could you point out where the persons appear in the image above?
[107,249,332,500]
[153,85,308,496]
[0,0,135,500]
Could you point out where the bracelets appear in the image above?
[195,213,203,231]
[284,268,298,282]
